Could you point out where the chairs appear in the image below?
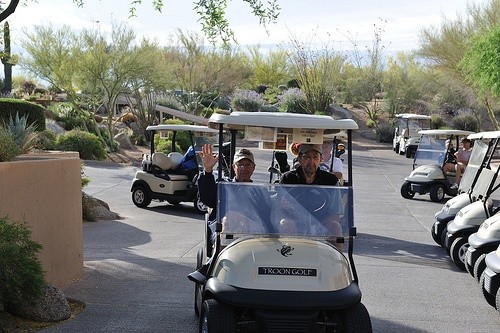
[151,151,192,181]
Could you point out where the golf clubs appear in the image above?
[268,158,300,179]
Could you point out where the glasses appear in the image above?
[301,156,319,160]
[235,163,254,168]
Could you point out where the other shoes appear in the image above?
[187,263,210,284]
[450,183,458,189]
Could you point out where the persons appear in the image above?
[187,141,345,284]
[178,137,205,181]
[443,138,473,189]
[436,139,456,166]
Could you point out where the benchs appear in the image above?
[447,170,465,177]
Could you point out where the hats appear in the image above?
[298,144,322,154]
[461,138,470,143]
[233,149,254,164]
